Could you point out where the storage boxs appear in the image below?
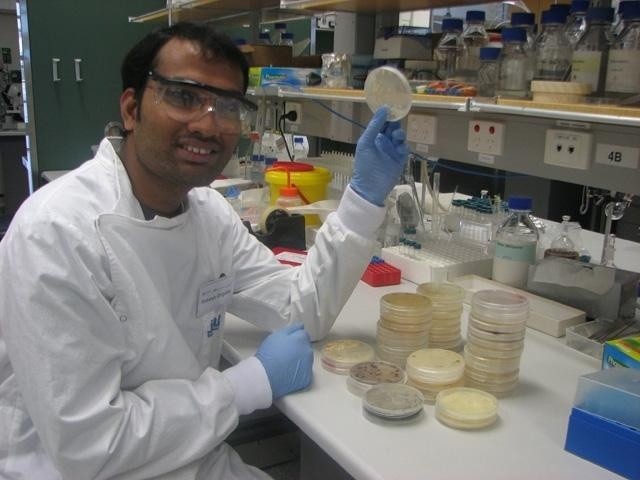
[236,42,294,66]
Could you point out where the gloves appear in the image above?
[254,319,315,399]
[349,106,411,207]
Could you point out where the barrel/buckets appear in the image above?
[264,161,332,227]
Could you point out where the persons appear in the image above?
[0,20,410,480]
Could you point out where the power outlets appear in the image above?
[468,120,506,155]
[285,101,301,125]
[405,112,437,146]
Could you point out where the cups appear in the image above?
[319,53,354,88]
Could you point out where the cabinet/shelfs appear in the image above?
[125,0,639,126]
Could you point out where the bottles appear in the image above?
[450,195,510,244]
[435,2,639,104]
[250,154,278,169]
[488,193,539,290]
[233,20,295,47]
[396,225,422,260]
[293,137,307,160]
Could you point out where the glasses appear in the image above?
[147,69,259,131]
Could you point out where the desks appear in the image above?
[41,168,639,480]
